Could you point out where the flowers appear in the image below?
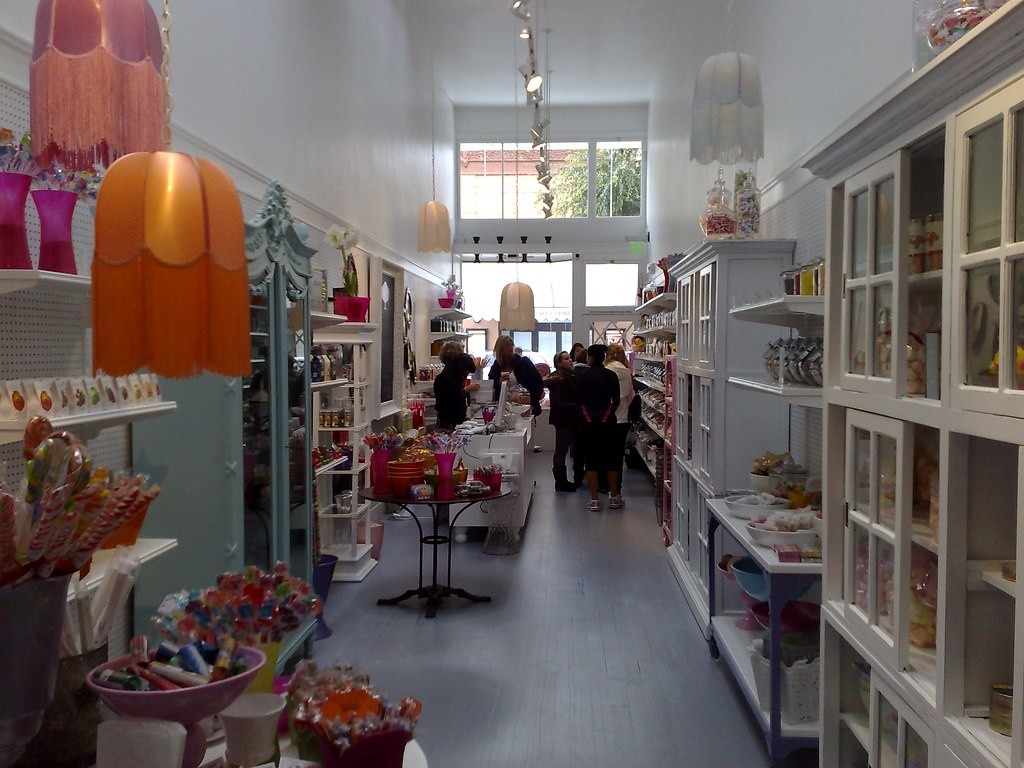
[324,226,365,296]
[442,276,465,300]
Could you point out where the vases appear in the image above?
[438,290,462,309]
[328,298,373,322]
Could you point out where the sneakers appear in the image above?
[586,499,601,511]
[609,496,625,508]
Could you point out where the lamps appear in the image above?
[91,146,251,383]
[30,0,172,179]
[497,1,554,331]
[691,1,767,164]
[415,44,454,251]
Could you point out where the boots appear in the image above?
[552,465,576,492]
[572,464,584,487]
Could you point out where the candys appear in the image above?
[290,658,419,755]
[928,4,1001,46]
[0,414,162,585]
[0,128,104,200]
[364,426,471,454]
[473,465,502,475]
[152,562,321,647]
[702,193,760,241]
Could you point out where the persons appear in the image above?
[489,346,531,365]
[542,343,635,511]
[489,336,546,430]
[433,340,480,432]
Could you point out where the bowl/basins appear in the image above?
[715,466,823,635]
[386,461,424,497]
[84,644,267,723]
[473,473,501,491]
[859,671,897,734]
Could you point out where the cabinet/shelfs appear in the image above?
[803,0,1024,768]
[628,293,677,487]
[0,270,180,607]
[667,238,801,636]
[424,309,473,362]
[316,340,375,582]
[131,178,319,690]
[412,373,470,424]
[700,294,829,768]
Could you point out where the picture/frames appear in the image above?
[369,255,405,419]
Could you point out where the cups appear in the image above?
[335,490,353,512]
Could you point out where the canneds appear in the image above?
[908,212,945,274]
[319,409,354,427]
[781,259,825,296]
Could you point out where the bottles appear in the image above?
[309,346,336,383]
[763,336,824,386]
[699,181,736,239]
[735,180,761,240]
[779,258,825,296]
[912,0,943,74]
[454,457,468,483]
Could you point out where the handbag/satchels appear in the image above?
[628,394,642,422]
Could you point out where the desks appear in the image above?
[358,485,512,619]
[198,701,428,768]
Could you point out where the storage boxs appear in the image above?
[747,639,824,724]
